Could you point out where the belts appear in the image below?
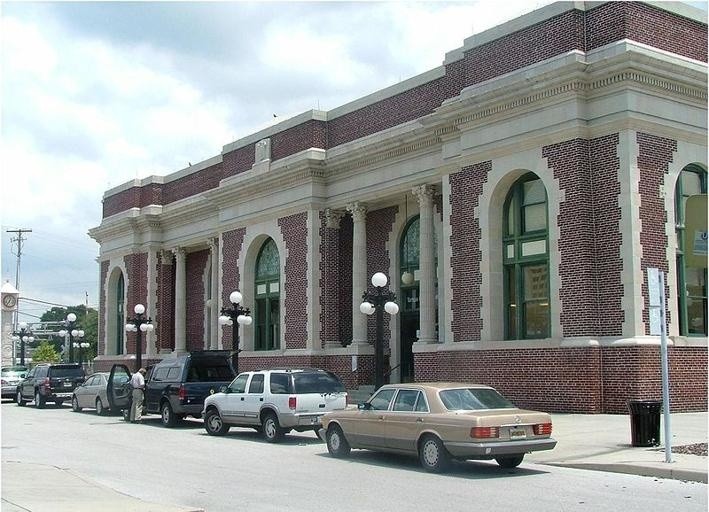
[134,388,142,389]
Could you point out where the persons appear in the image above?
[130,367,147,422]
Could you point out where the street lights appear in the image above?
[73,339,90,362]
[125,303,154,372]
[58,312,84,362]
[10,320,35,367]
[218,290,254,376]
[359,272,402,391]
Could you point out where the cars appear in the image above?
[315,381,559,474]
[70,371,135,416]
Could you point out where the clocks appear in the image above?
[2,294,18,309]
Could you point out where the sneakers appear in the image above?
[130,420,136,424]
[136,420,146,424]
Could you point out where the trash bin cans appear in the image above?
[625,399,662,447]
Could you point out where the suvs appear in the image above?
[1,366,28,399]
[14,362,87,408]
[201,366,348,445]
[106,349,243,427]
